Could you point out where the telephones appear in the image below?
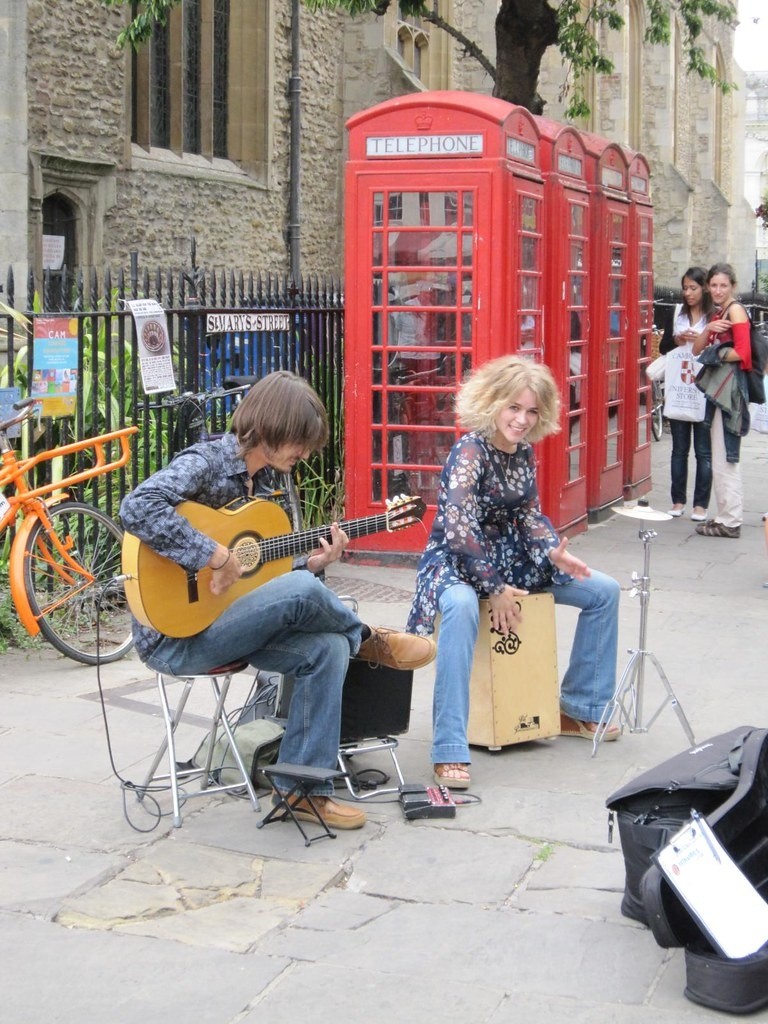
[375,273,415,370]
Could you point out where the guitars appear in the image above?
[119,490,427,641]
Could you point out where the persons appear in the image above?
[120,371,437,829]
[659,263,751,538]
[406,355,621,789]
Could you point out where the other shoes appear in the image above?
[698,519,718,524]
[667,506,686,518]
[696,525,741,539]
[691,507,709,521]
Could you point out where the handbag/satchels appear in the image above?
[718,326,768,405]
[646,356,665,383]
[605,726,759,923]
[664,345,707,424]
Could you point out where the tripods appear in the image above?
[591,520,696,758]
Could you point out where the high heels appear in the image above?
[432,763,472,789]
[560,710,622,741]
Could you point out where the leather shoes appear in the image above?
[360,625,439,673]
[272,784,368,831]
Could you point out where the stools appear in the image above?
[132,656,262,828]
[434,592,558,751]
[258,763,350,845]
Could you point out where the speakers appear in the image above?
[340,659,414,744]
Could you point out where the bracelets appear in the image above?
[209,549,230,570]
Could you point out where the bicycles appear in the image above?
[0,394,142,666]
[136,377,306,559]
[652,380,664,442]
